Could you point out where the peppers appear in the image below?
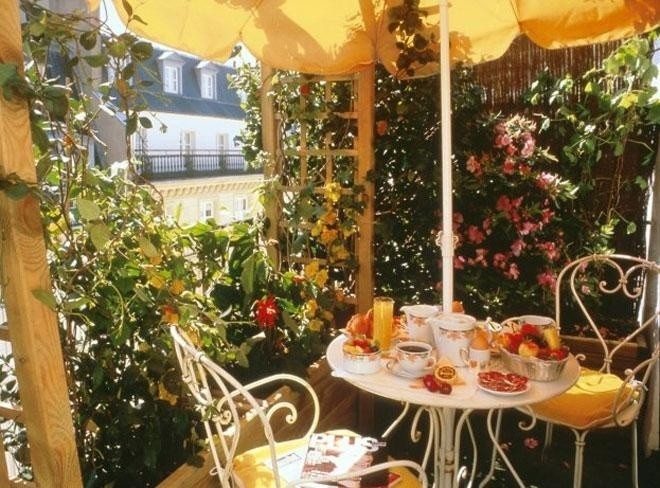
[505,324,570,361]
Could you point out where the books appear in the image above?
[263,433,402,488]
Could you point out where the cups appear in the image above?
[372,296,395,356]
[340,303,571,395]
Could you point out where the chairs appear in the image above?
[170,320,433,487]
[479,254,660,486]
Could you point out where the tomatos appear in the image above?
[423,375,452,394]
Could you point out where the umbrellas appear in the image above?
[113,0,660,488]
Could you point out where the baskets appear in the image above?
[497,342,570,381]
